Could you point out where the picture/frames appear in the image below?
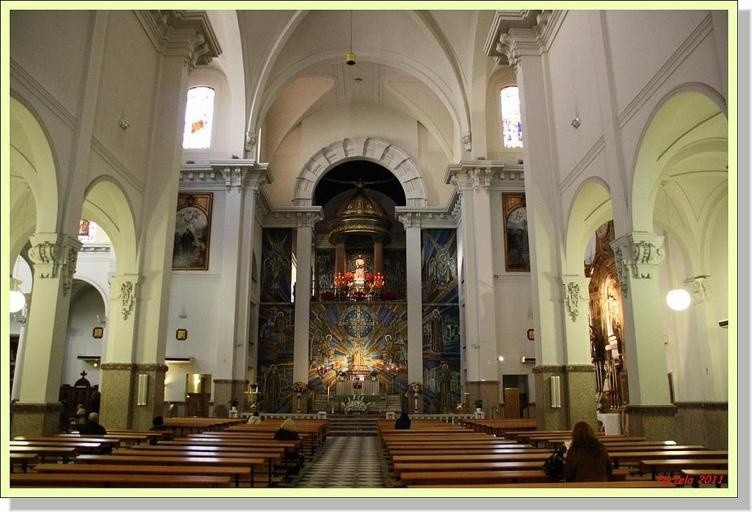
[169,191,214,271]
[500,190,531,272]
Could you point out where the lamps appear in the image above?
[345,11,363,71]
[664,275,709,316]
[328,255,390,304]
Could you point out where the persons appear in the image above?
[385,334,394,363]
[87,392,100,412]
[78,412,113,455]
[431,309,443,352]
[264,365,280,408]
[70,404,85,424]
[437,361,451,403]
[248,412,261,425]
[322,333,332,357]
[147,415,169,444]
[394,412,411,429]
[562,422,613,482]
[274,418,299,460]
[355,254,365,281]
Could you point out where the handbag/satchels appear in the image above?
[543,455,564,477]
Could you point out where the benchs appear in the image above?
[10,410,729,489]
